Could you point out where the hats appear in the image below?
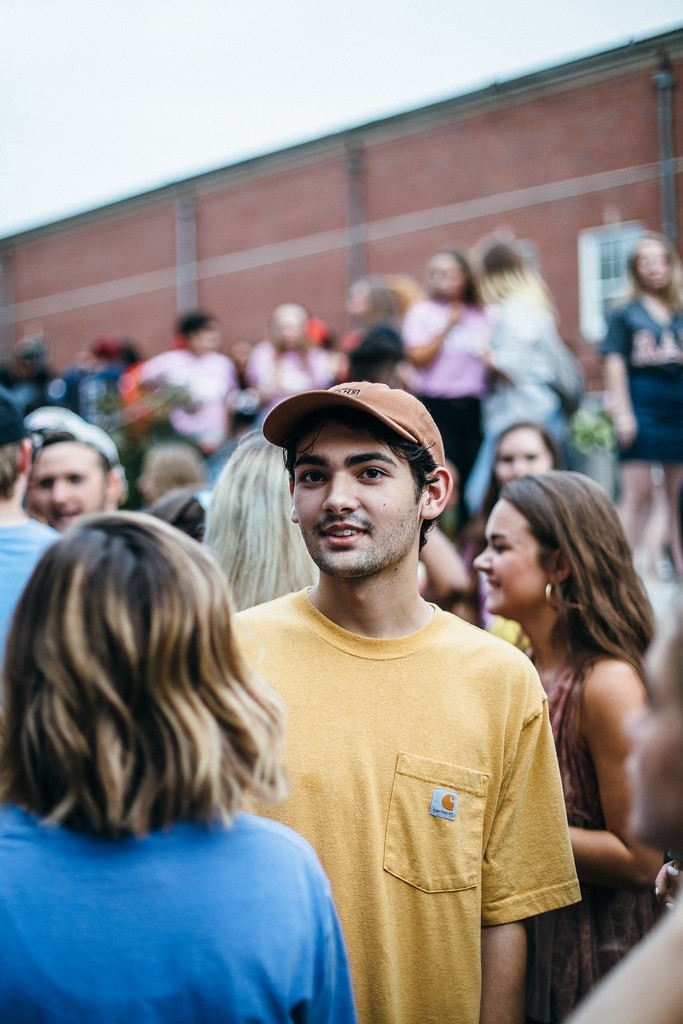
[261,380,447,470]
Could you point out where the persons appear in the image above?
[230,382,581,1024]
[472,471,683,1024]
[0,232,683,658]
[0,511,358,1024]
